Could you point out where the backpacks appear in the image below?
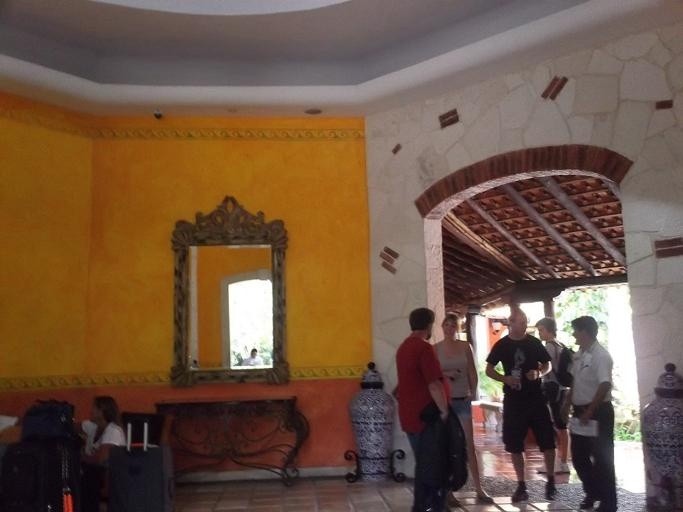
[545,340,575,388]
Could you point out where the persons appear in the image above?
[80,395,127,511]
[241,348,263,366]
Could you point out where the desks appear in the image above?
[148,392,310,500]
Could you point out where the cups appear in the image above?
[511,368,522,380]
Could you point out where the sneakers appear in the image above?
[447,497,466,509]
[476,491,494,503]
[511,482,529,503]
[595,501,619,511]
[579,487,599,509]
[545,479,557,500]
[536,460,571,474]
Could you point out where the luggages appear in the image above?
[112,443,176,512]
[0,438,81,511]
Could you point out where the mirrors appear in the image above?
[167,192,297,388]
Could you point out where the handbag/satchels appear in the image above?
[21,399,75,438]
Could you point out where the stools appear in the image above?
[477,401,503,433]
